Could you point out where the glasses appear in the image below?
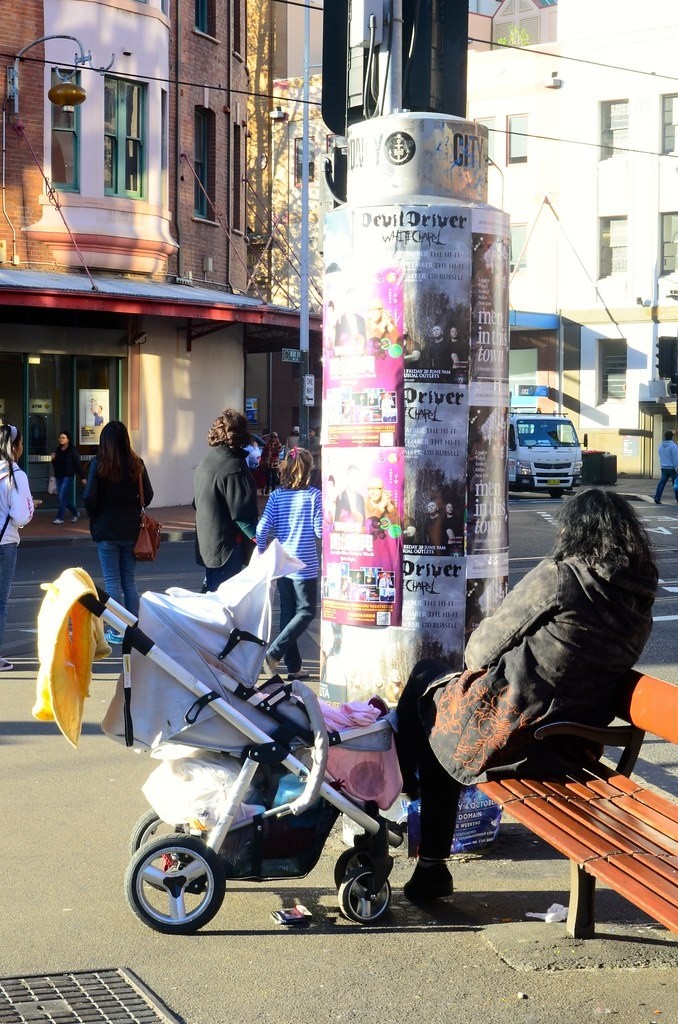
[271,436,277,438]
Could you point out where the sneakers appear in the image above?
[103,629,124,644]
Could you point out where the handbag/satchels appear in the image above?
[142,748,255,827]
[131,513,163,560]
[407,785,502,858]
[672,476,678,491]
[48,476,58,495]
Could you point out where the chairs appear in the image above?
[534,425,551,441]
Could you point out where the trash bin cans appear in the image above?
[598,454,617,485]
[580,451,602,484]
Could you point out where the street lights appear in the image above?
[267,105,290,305]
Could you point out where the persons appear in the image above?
[423,501,463,547]
[0,424,42,671]
[341,568,393,601]
[193,410,323,680]
[654,430,678,504]
[326,300,399,357]
[324,465,398,534]
[399,488,659,898]
[90,399,104,426]
[83,421,154,645]
[51,431,86,524]
[359,389,394,417]
[255,685,404,811]
[405,325,471,371]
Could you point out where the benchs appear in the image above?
[474,667,678,941]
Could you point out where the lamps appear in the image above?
[5,34,116,116]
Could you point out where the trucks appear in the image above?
[507,410,588,499]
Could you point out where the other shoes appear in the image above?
[401,775,422,801]
[0,656,13,670]
[654,499,662,504]
[263,655,280,678]
[71,511,80,522]
[288,668,309,681]
[261,490,265,496]
[403,864,454,898]
[265,487,270,494]
[53,518,64,524]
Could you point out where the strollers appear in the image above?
[72,585,410,935]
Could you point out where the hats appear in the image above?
[293,426,300,434]
